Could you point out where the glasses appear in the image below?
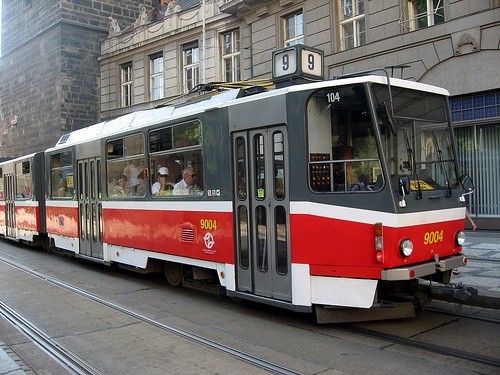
[186,173,197,178]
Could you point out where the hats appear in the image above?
[158,167,169,176]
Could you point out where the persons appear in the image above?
[151,168,174,195]
[172,167,204,196]
[112,174,127,196]
[24,185,32,198]
[57,179,73,197]
[122,158,145,187]
[135,169,145,196]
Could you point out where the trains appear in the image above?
[1,44,476,323]
